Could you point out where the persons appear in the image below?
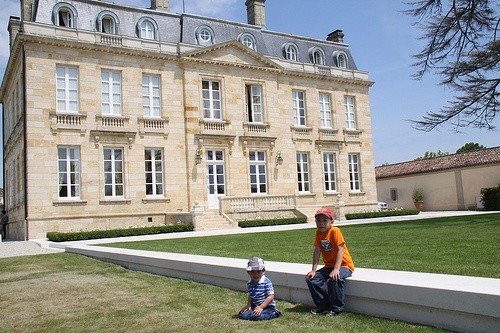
[305,208,354,317]
[238,257,283,321]
[0,210,8,240]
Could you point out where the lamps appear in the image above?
[276,151,283,165]
[194,148,202,164]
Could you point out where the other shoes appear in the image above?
[311,308,332,315]
[274,309,282,318]
[326,310,344,317]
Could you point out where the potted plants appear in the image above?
[411,187,424,212]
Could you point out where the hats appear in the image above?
[245,257,265,271]
[315,208,334,219]
